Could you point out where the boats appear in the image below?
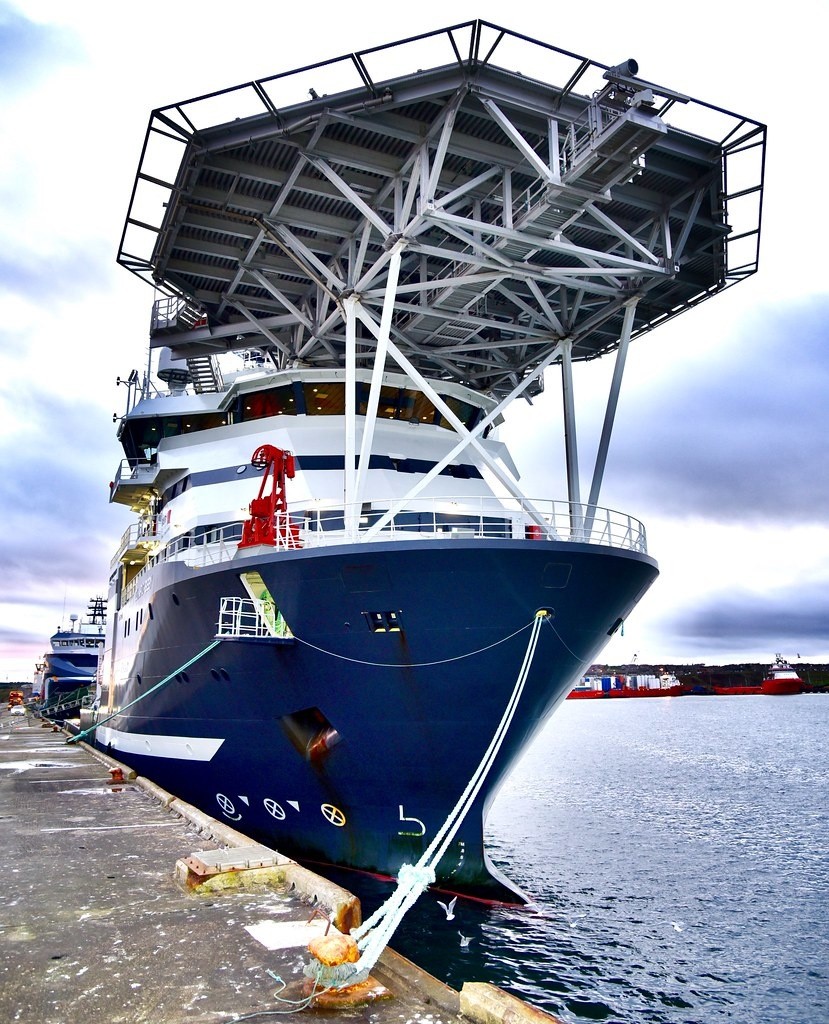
[713,652,805,695]
[64,19,766,910]
[35,595,108,721]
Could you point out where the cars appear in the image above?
[11,705,25,716]
